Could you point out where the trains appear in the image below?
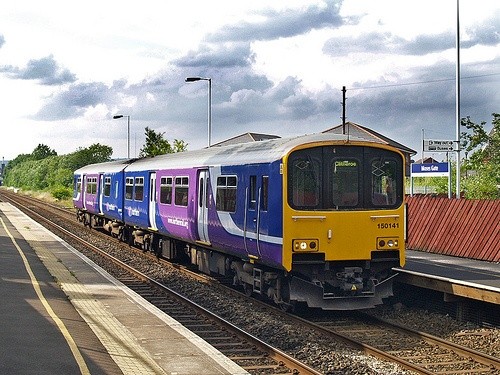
[71,125,410,312]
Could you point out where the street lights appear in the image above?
[185,76,213,152]
[113,114,132,160]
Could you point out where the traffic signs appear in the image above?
[422,139,455,152]
